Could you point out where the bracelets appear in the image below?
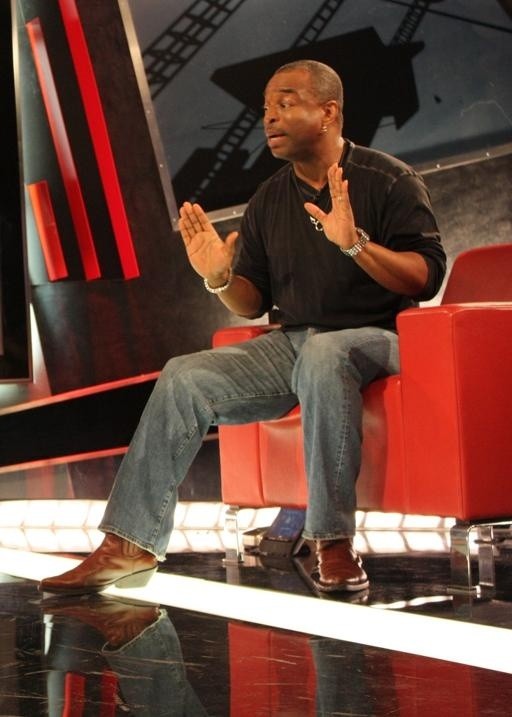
[204,267,235,293]
[340,227,370,257]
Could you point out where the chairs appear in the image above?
[211,240,512,612]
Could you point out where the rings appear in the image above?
[336,196,342,204]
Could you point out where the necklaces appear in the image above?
[290,142,346,233]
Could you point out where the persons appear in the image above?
[40,62,446,596]
[44,598,373,716]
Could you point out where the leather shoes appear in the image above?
[315,538,370,592]
[36,532,156,596]
